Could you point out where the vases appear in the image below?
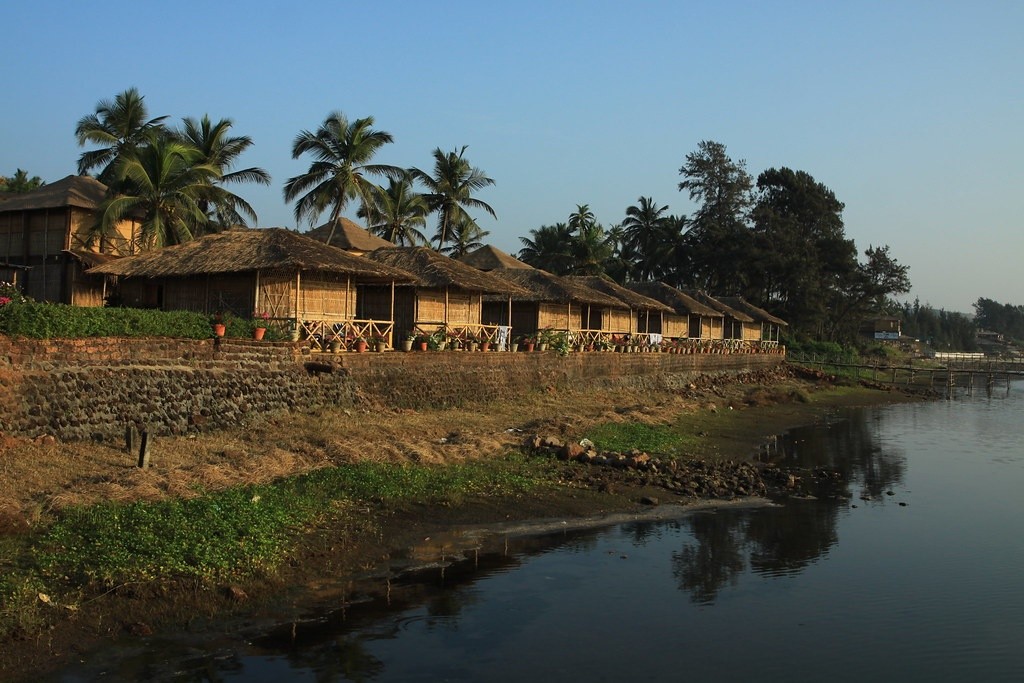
[617,346,624,354]
[491,344,502,352]
[640,347,645,353]
[467,343,475,351]
[376,342,386,352]
[449,342,459,351]
[666,347,763,354]
[649,346,654,353]
[525,344,534,352]
[625,346,631,353]
[369,343,374,351]
[328,342,342,353]
[252,327,266,340]
[655,347,662,352]
[402,340,413,352]
[215,324,226,337]
[438,341,446,350]
[631,346,638,352]
[347,340,353,352]
[479,343,489,351]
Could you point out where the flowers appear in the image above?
[429,332,444,351]
[664,341,726,348]
[404,329,417,340]
[365,332,389,343]
[347,333,356,340]
[251,311,270,327]
[447,331,490,343]
[617,339,660,347]
[212,311,233,325]
[524,334,536,344]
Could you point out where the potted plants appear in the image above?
[287,320,299,342]
[577,338,585,352]
[538,328,568,357]
[595,341,604,352]
[766,342,780,354]
[586,338,594,351]
[604,343,616,352]
[355,337,365,352]
[509,336,521,352]
[419,337,429,351]
